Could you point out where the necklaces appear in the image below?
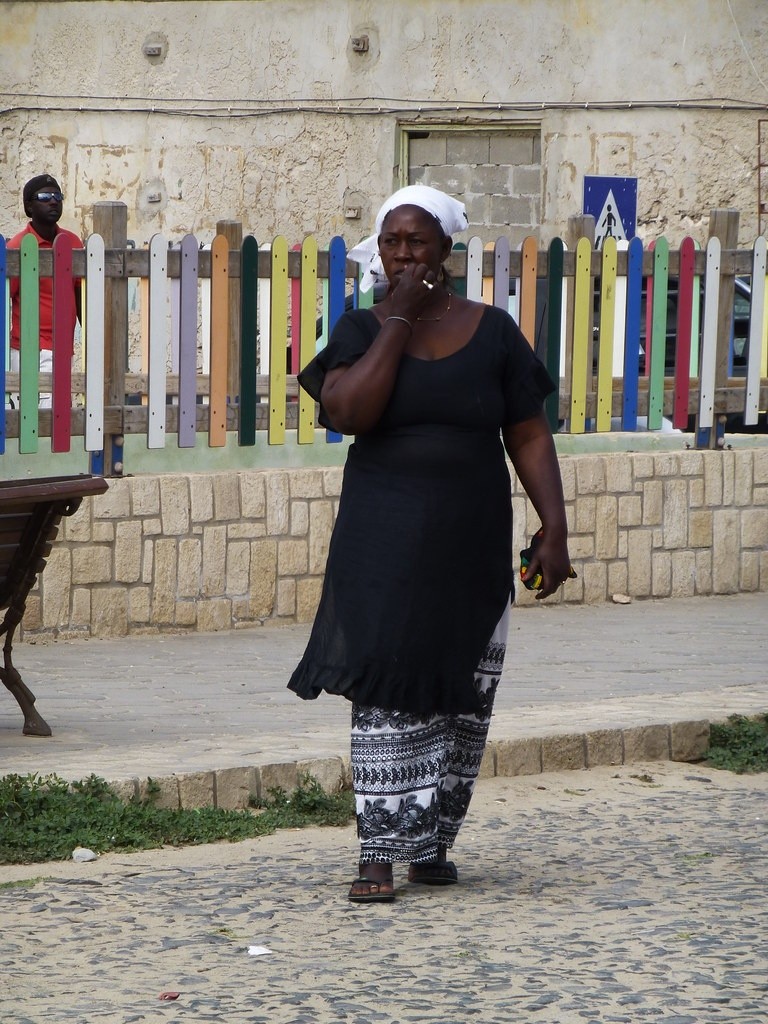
[417,292,451,321]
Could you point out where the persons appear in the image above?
[7,174,84,409]
[287,185,577,902]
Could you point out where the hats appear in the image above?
[23,175,61,218]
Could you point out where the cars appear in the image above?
[286,276,753,378]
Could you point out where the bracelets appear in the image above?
[385,317,412,334]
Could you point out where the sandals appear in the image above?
[408,858,459,883]
[347,877,395,902]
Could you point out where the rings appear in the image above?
[562,581,565,583]
[423,280,433,290]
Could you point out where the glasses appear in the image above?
[28,192,65,202]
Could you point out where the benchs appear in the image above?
[0,474,110,737]
